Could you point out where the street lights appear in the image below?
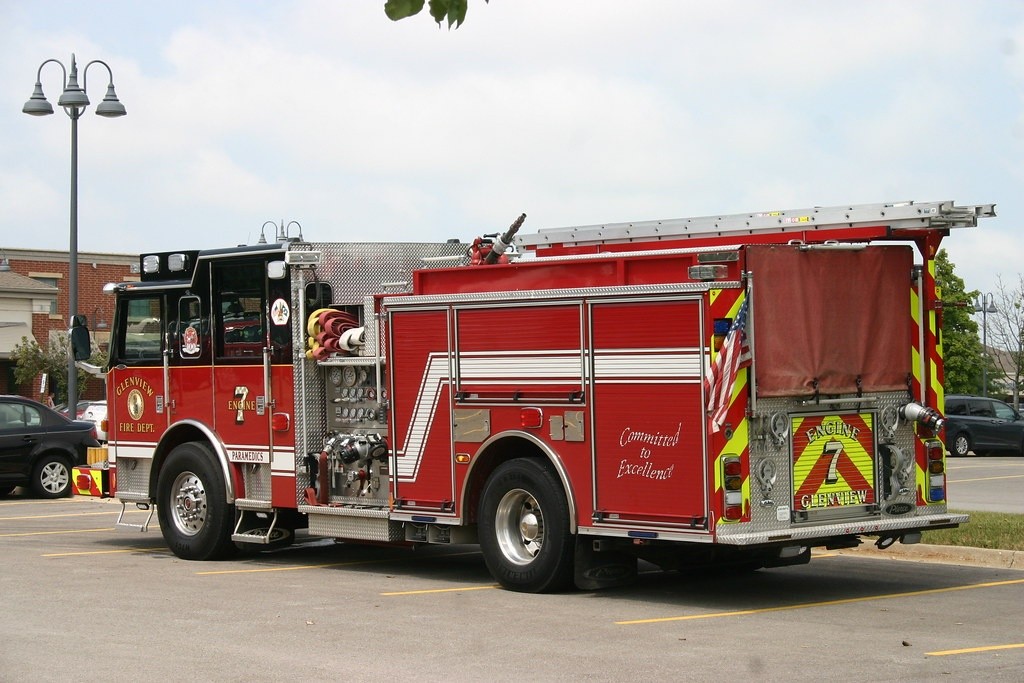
[23,53,127,420]
[973,292,998,397]
[93,307,107,340]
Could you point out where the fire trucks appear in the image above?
[69,199,998,594]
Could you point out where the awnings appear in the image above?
[0,321,43,358]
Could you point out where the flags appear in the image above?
[704,296,753,436]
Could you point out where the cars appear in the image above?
[32,400,92,426]
[942,394,1024,458]
[0,402,41,428]
[81,400,108,445]
[0,395,102,499]
[995,403,1024,420]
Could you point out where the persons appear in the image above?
[47,392,55,407]
